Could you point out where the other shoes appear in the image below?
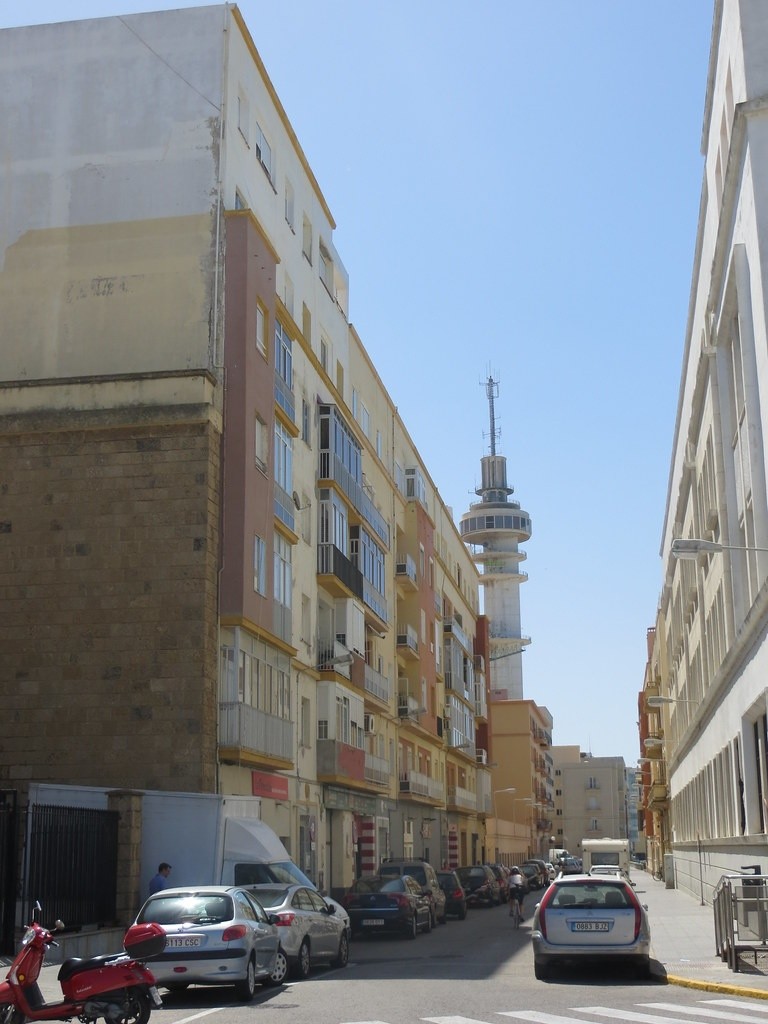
[509,910,513,917]
[520,916,525,922]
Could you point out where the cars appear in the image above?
[452,837,637,906]
[124,885,290,1002]
[338,874,433,940]
[232,882,351,979]
[434,870,472,921]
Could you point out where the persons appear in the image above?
[507,866,526,922]
[149,863,172,897]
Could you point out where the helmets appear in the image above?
[511,866,519,873]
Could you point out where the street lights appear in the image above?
[292,653,354,868]
[515,796,542,839]
[492,787,515,836]
[385,707,430,858]
[444,741,474,867]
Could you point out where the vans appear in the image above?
[375,855,447,930]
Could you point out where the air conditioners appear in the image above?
[476,747,488,765]
[364,713,375,733]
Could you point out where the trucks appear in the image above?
[15,781,354,948]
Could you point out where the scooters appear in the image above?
[0,900,170,1024]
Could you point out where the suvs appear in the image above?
[524,872,653,982]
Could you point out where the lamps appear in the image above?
[670,539,768,560]
[632,738,662,789]
[438,743,471,753]
[297,654,354,683]
[495,787,542,809]
[387,707,427,729]
[646,697,699,707]
